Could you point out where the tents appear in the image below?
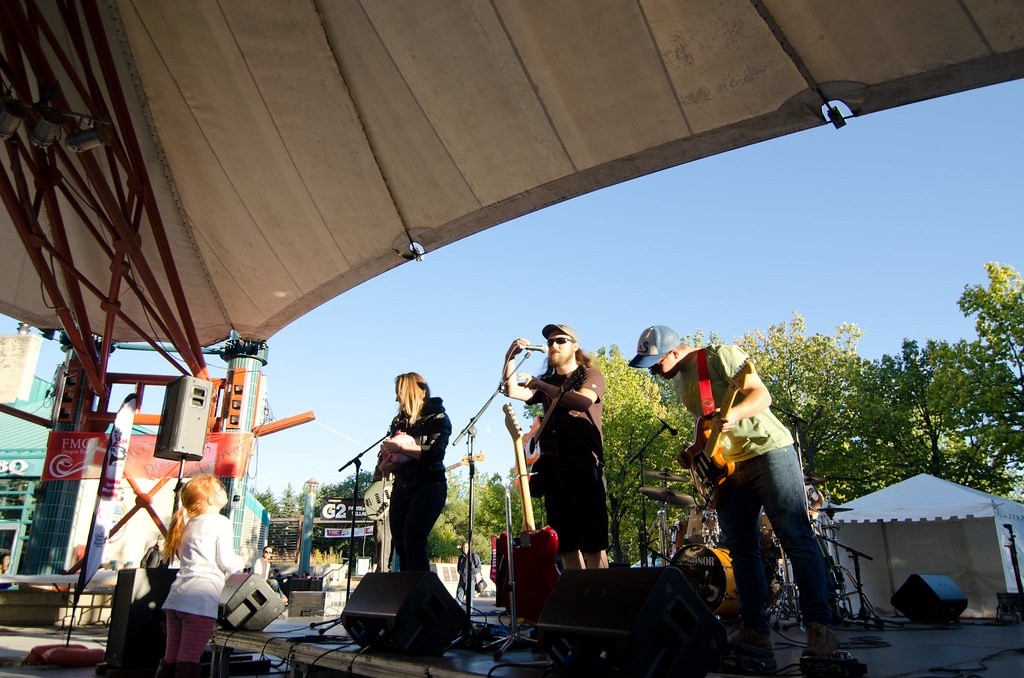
[827,473,1024,615]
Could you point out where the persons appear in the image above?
[455,541,481,606]
[378,372,452,570]
[503,323,610,568]
[254,546,274,579]
[155,473,247,678]
[628,325,839,655]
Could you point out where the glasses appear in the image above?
[264,551,271,554]
[648,350,670,372]
[206,484,227,499]
[547,338,572,347]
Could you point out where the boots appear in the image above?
[155,659,175,678]
[174,661,201,678]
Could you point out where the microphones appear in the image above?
[520,344,548,353]
[657,417,678,436]
[701,588,711,598]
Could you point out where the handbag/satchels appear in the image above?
[475,579,487,593]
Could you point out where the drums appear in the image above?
[672,521,688,548]
[668,541,744,619]
[683,505,720,545]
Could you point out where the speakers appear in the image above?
[340,572,470,657]
[890,573,968,625]
[216,572,286,632]
[104,568,181,672]
[535,565,727,677]
[154,376,213,462]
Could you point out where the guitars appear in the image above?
[514,362,587,500]
[500,403,564,620]
[686,359,754,509]
[363,450,487,521]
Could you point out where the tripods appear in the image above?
[483,472,538,660]
[767,511,883,631]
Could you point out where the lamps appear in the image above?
[16,322,31,335]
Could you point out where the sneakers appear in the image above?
[723,621,772,651]
[801,621,840,657]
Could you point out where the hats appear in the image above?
[628,325,680,368]
[542,324,577,343]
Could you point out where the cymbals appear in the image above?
[803,475,829,486]
[640,469,689,482]
[816,505,855,517]
[639,487,696,506]
